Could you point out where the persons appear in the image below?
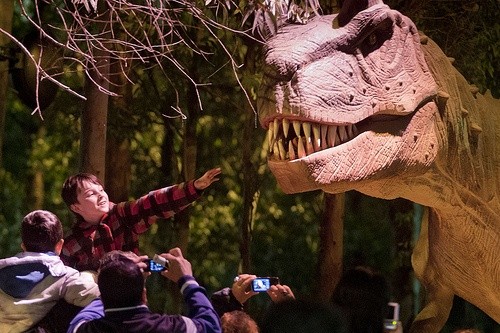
[209,248,404,333]
[0,168,222,333]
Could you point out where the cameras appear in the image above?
[252,277,280,292]
[383,303,400,329]
[145,258,169,271]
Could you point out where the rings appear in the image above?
[279,291,290,296]
[233,276,240,283]
